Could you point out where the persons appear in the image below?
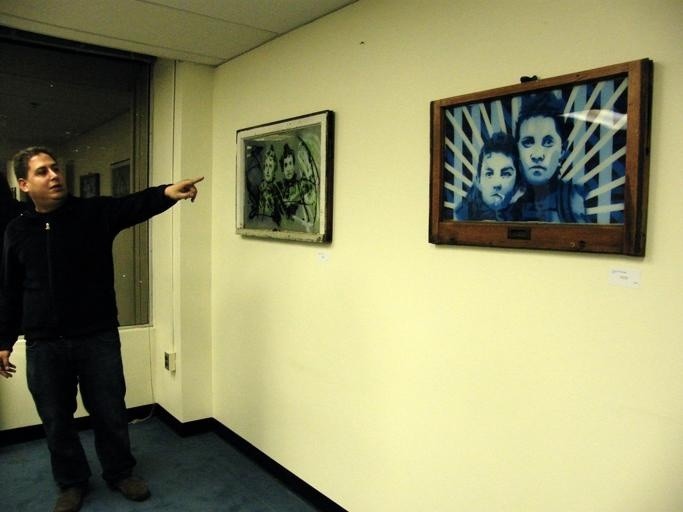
[455,93,587,223]
[258,151,316,225]
[0,147,205,512]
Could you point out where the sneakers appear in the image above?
[107,475,151,502]
[53,480,90,512]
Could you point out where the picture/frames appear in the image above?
[80,174,100,198]
[109,159,130,197]
[235,110,335,243]
[429,57,647,257]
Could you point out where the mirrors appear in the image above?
[0,24,154,335]
